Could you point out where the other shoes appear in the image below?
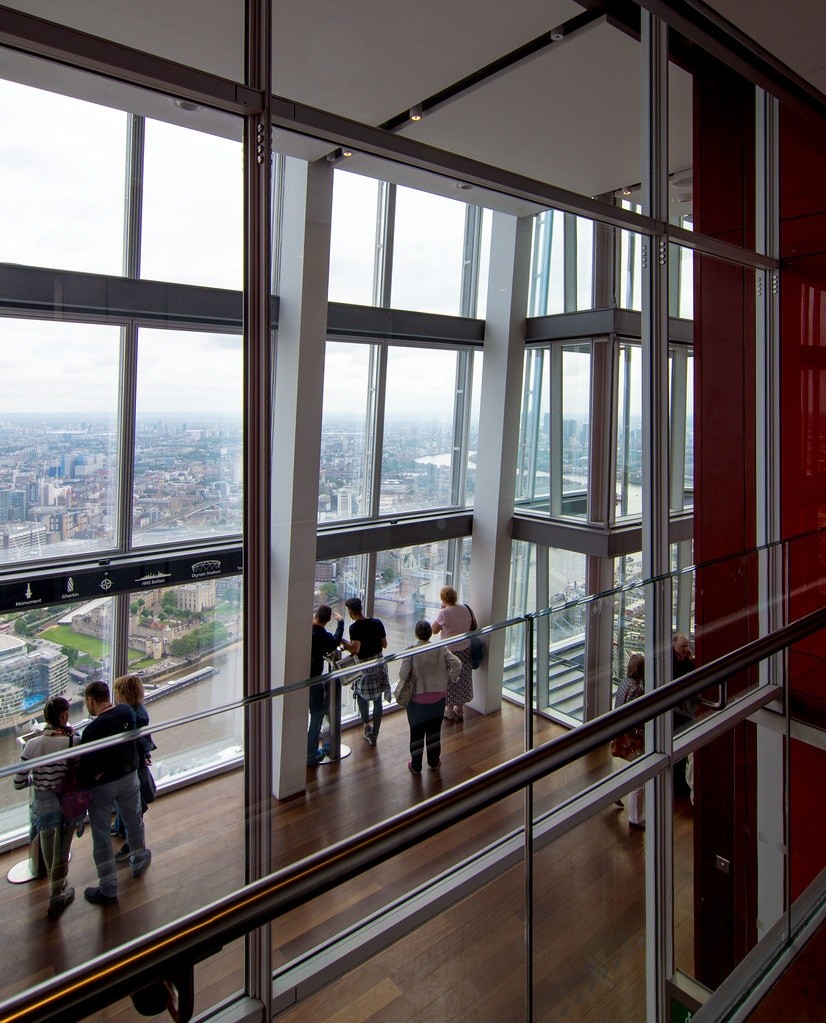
[628,821,646,831]
[365,726,373,734]
[444,710,455,718]
[115,845,132,861]
[455,708,465,721]
[85,887,118,907]
[363,730,378,745]
[133,849,151,876]
[309,749,323,761]
[47,888,75,914]
[408,759,421,774]
[431,762,442,769]
[109,825,116,833]
[59,879,67,892]
[614,799,625,806]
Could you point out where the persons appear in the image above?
[14,676,158,916]
[307,585,474,761]
[613,632,695,828]
[398,621,463,774]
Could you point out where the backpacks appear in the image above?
[59,736,93,819]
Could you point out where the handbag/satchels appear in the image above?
[464,603,487,669]
[394,644,417,706]
[611,733,646,762]
[139,767,157,803]
